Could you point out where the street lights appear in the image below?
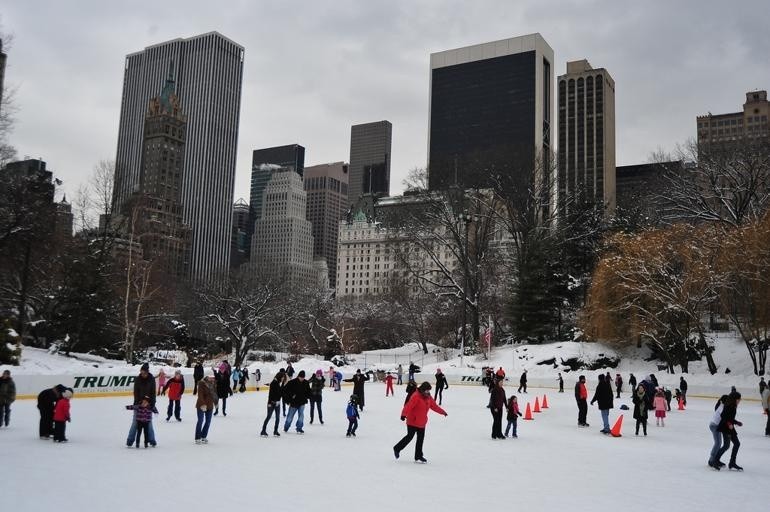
[459,209,479,348]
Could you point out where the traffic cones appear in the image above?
[609,413,623,437]
[677,397,686,410]
[542,393,548,409]
[523,402,534,420]
[532,396,542,413]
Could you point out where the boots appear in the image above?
[393,445,400,459]
[491,421,742,471]
[415,456,427,462]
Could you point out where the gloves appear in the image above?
[401,416,407,422]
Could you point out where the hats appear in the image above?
[140,362,322,382]
[62,389,73,398]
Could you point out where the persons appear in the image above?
[0,369,17,428]
[232,363,250,392]
[708,385,744,470]
[192,359,234,444]
[574,371,623,433]
[260,361,324,438]
[382,361,414,398]
[481,366,522,439]
[34,383,74,439]
[759,377,769,436]
[342,369,370,438]
[555,372,565,393]
[518,368,529,393]
[326,367,342,391]
[125,362,160,448]
[51,389,73,445]
[156,367,185,421]
[629,373,687,436]
[392,368,449,465]
[252,369,262,391]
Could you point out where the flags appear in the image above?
[482,321,491,347]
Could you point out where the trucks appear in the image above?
[148,350,188,367]
